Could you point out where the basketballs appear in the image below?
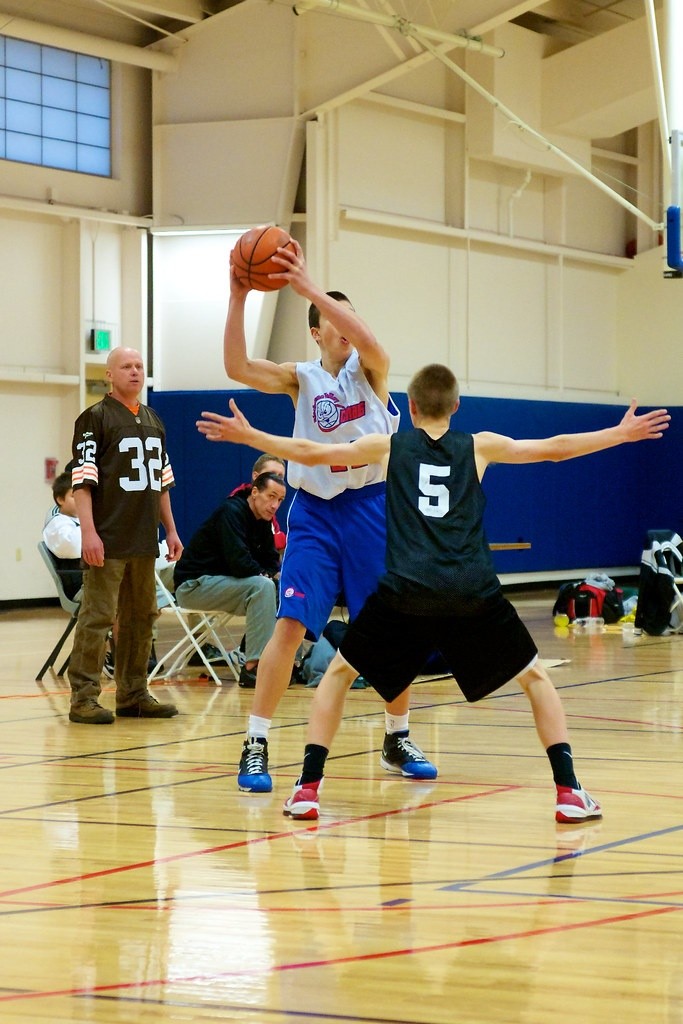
[232,226,295,293]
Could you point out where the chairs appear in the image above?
[147,555,243,687]
[648,529,683,636]
[36,542,117,681]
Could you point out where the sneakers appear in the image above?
[238,663,296,688]
[116,694,178,718]
[556,781,603,824]
[237,734,272,793]
[147,651,165,674]
[68,699,114,723]
[101,651,115,679]
[229,645,247,668]
[186,642,234,666]
[379,730,437,780]
[283,778,324,821]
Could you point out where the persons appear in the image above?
[42,472,230,679]
[173,471,286,687]
[67,347,185,723]
[229,454,287,651]
[195,366,673,821]
[224,245,440,792]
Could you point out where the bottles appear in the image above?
[572,617,604,629]
[554,613,570,625]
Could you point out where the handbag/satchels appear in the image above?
[551,579,625,624]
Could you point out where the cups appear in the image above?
[623,623,635,645]
